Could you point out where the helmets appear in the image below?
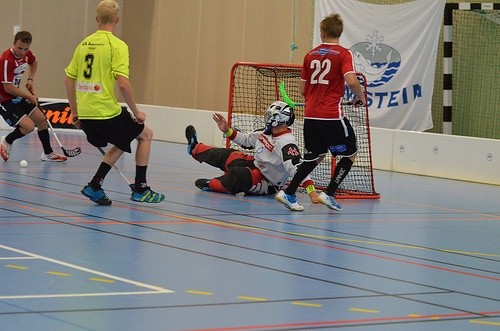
[265,101,295,127]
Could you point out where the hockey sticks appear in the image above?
[26,83,81,157]
[73,117,133,188]
[279,81,363,108]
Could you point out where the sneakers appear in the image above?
[185,125,197,155]
[195,179,213,192]
[41,153,67,161]
[0,136,9,161]
[318,192,343,210]
[276,190,304,210]
[131,188,165,203]
[81,184,112,205]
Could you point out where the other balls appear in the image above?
[20,160,28,167]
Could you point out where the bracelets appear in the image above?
[28,78,33,81]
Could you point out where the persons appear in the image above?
[0,31,67,162]
[64,0,166,206]
[274,14,366,212]
[185,101,320,203]
